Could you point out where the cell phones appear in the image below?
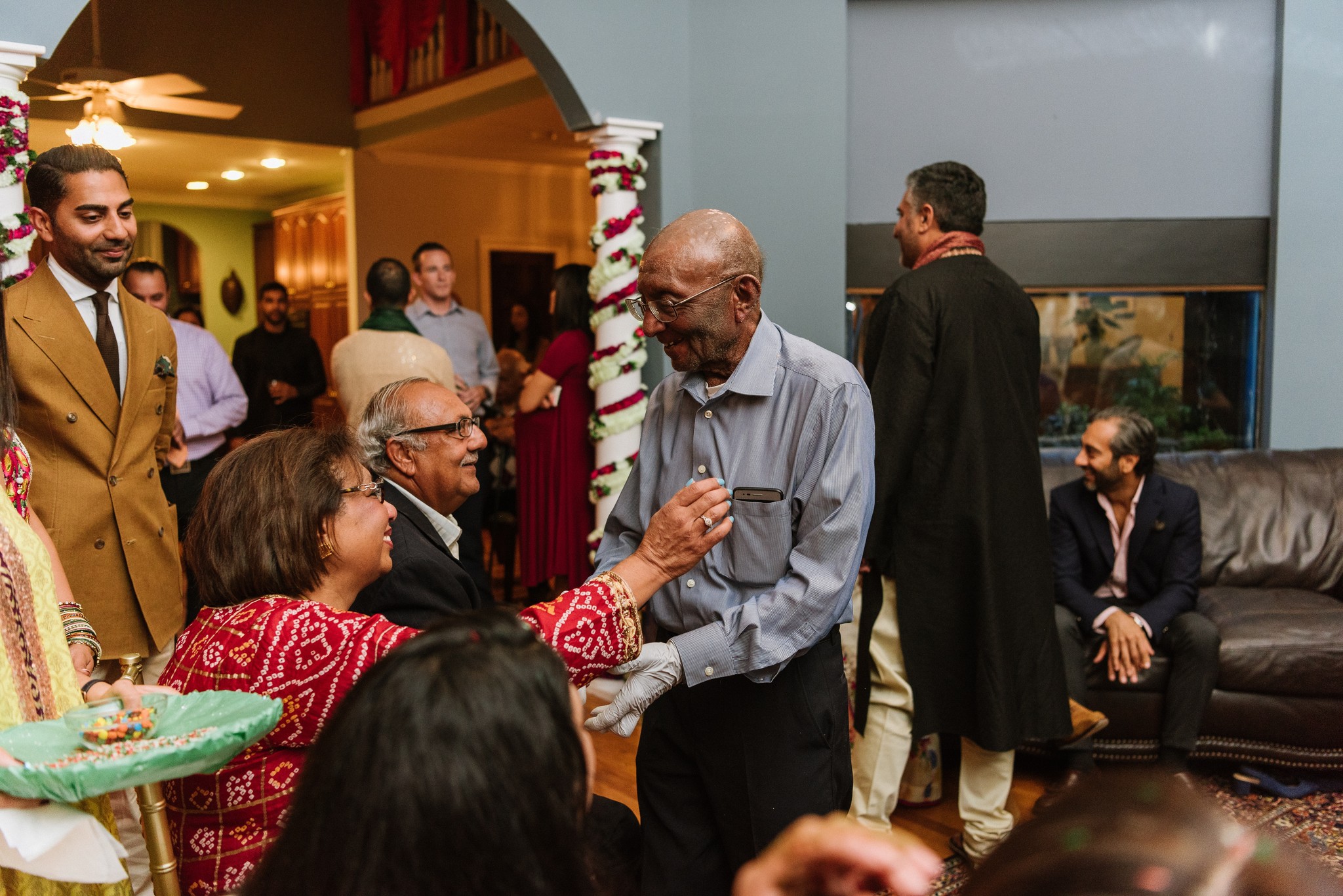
[539,385,562,407]
[732,487,784,503]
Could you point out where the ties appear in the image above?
[165,407,187,469]
[88,292,120,409]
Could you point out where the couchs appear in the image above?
[1039,445,1343,780]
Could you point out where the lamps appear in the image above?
[31,1,240,151]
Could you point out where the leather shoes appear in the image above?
[1032,767,1106,815]
[1170,769,1205,801]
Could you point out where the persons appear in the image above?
[168,305,207,330]
[0,464,182,896]
[330,256,457,456]
[351,378,488,621]
[515,261,598,605]
[225,279,327,455]
[1031,405,1223,814]
[228,607,600,894]
[492,296,552,368]
[0,140,188,834]
[133,421,735,896]
[578,206,878,896]
[120,255,250,626]
[401,241,501,611]
[837,161,1112,869]
[0,285,102,678]
[477,346,552,607]
[727,769,1343,896]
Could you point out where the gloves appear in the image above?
[582,641,683,739]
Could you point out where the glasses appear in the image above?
[625,275,740,322]
[337,477,385,504]
[396,417,480,437]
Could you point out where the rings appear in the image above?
[698,514,713,530]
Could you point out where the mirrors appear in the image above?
[846,277,1266,450]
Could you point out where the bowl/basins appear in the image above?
[65,690,169,750]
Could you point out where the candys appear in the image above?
[78,707,159,746]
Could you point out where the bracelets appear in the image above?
[79,679,113,706]
[57,602,103,671]
